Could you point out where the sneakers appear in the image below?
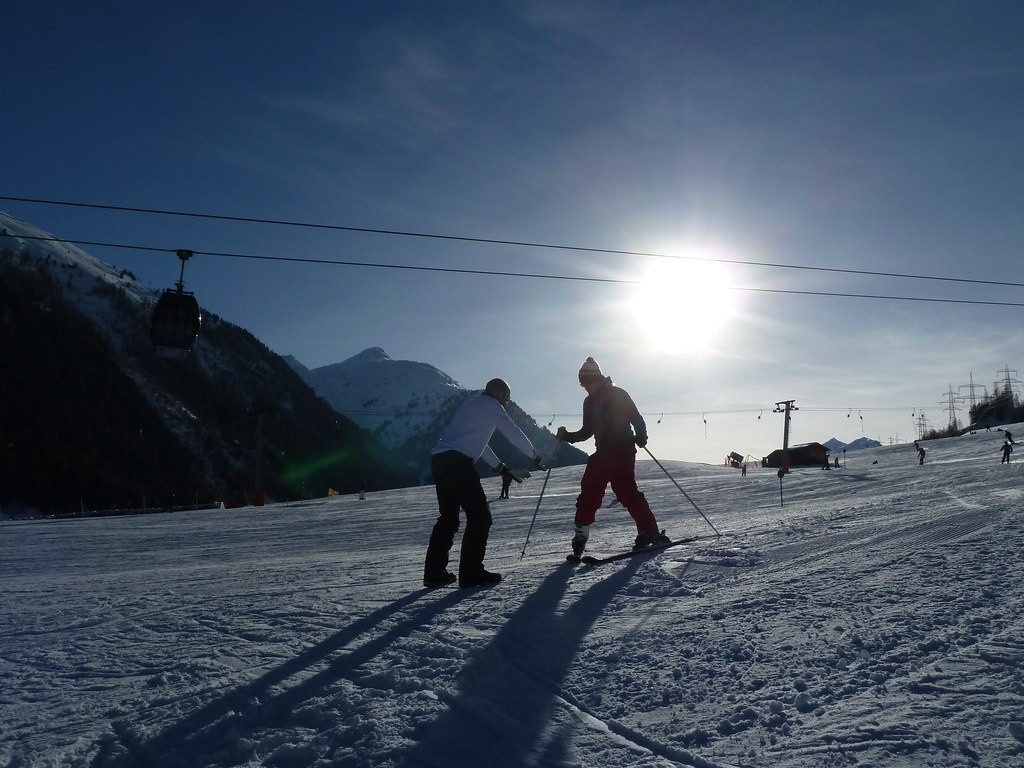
[459,570,502,588]
[422,573,457,589]
[632,529,671,552]
[572,525,589,556]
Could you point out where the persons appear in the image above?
[968,423,1004,434]
[918,448,925,465]
[555,356,660,562]
[835,457,840,468]
[1000,441,1013,464]
[822,454,830,470]
[498,465,513,500]
[741,465,747,476]
[872,460,878,464]
[423,378,547,589]
[913,441,920,451]
[1004,429,1016,445]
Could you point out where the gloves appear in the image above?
[633,435,648,448]
[492,461,531,483]
[556,426,571,443]
[528,449,554,471]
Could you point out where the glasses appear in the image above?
[580,381,597,387]
[505,395,511,401]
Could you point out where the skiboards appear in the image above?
[565,534,700,565]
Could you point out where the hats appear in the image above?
[578,357,601,382]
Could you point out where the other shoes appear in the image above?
[499,496,509,500]
